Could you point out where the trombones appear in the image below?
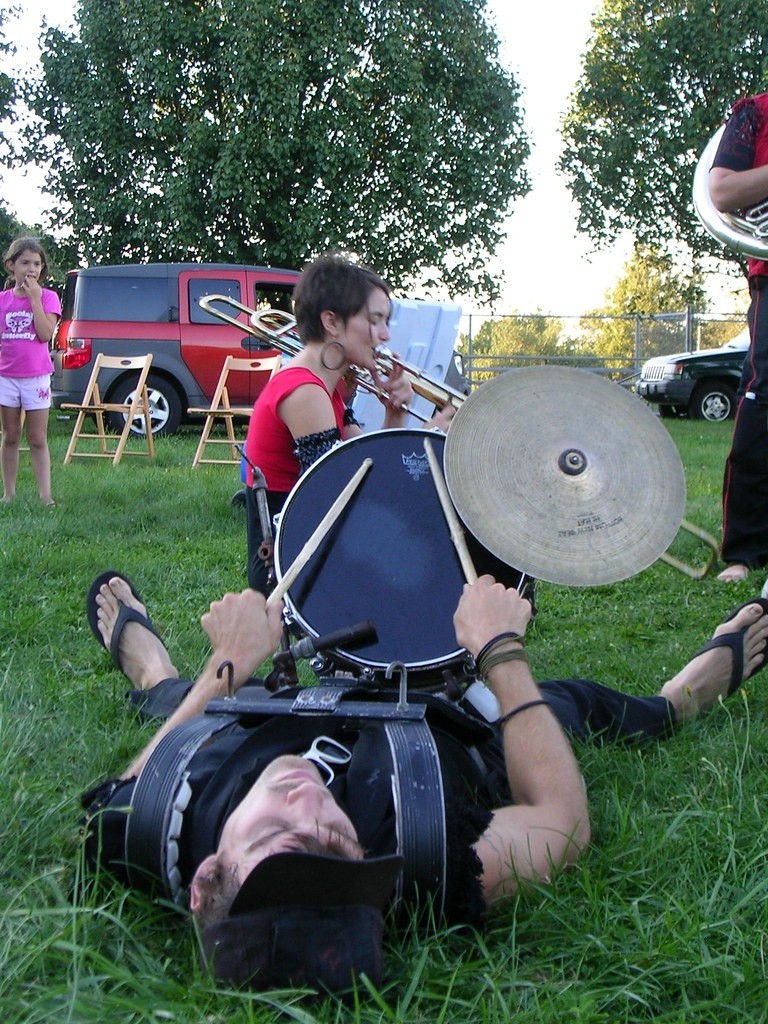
[194,290,722,584]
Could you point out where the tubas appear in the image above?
[691,108,768,264]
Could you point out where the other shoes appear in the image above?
[718,564,748,583]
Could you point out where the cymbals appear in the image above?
[442,364,688,591]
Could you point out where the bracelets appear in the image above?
[476,632,529,679]
[498,700,552,730]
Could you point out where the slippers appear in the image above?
[86,570,166,690]
[688,596,768,700]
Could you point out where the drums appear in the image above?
[265,428,538,694]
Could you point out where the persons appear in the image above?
[708,92,768,582]
[245,259,458,602]
[0,238,63,507]
[84,570,768,1006]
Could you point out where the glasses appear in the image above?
[301,735,351,787]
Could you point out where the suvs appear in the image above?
[49,262,303,440]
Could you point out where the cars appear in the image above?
[635,322,751,423]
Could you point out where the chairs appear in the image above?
[60,352,156,469]
[187,356,283,472]
